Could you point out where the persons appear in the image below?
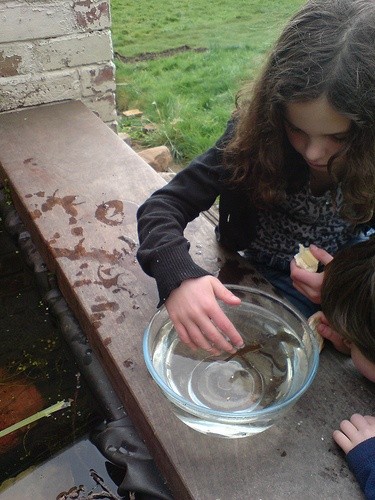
[316,236,375,500]
[134,1,372,359]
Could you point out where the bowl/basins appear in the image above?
[142,284,319,440]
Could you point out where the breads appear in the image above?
[301,317,325,355]
[294,243,319,273]
